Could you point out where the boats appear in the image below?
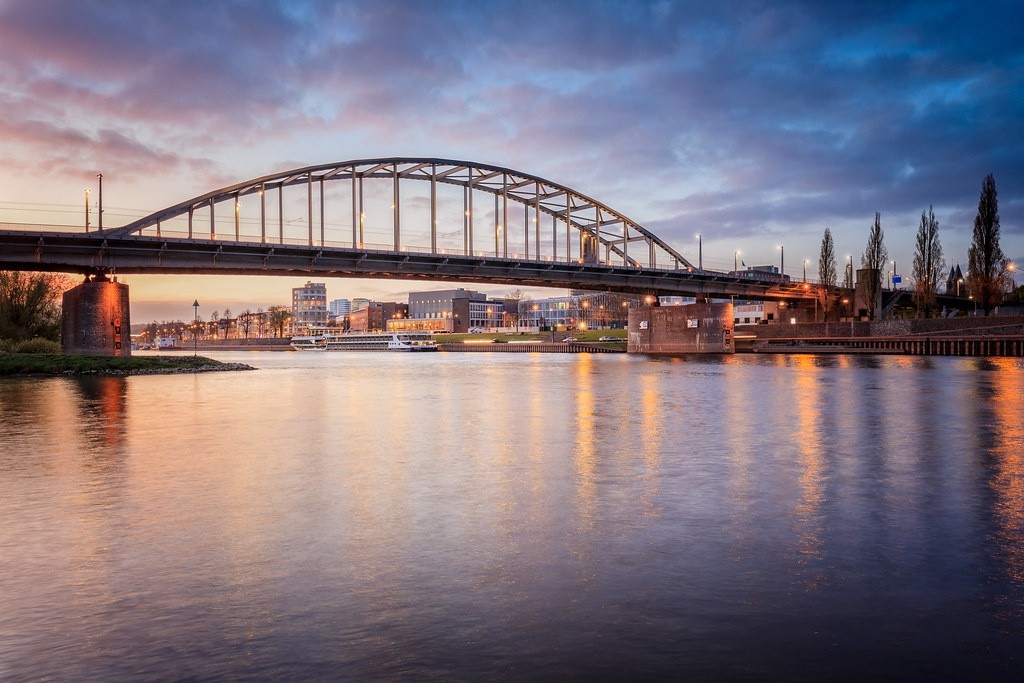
[131,336,182,351]
[289,322,438,352]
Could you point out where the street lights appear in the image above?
[780,245,784,280]
[804,260,808,285]
[84,188,92,233]
[697,234,703,271]
[891,260,896,290]
[888,271,894,291]
[97,173,103,232]
[848,255,853,289]
[734,250,740,277]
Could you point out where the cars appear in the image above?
[563,336,578,342]
[599,335,622,341]
